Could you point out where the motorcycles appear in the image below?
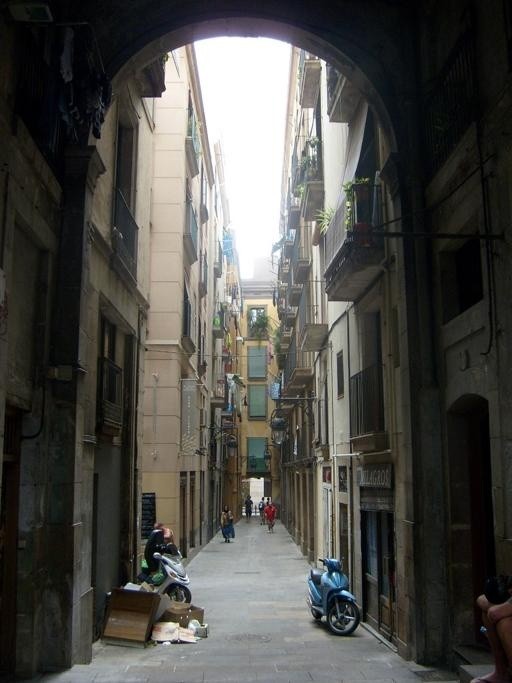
[306,555,360,635]
[141,527,192,603]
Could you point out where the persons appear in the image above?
[244,495,253,518]
[470,588,512,683]
[220,504,233,543]
[144,522,174,575]
[259,497,277,533]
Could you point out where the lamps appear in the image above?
[270,396,314,446]
[208,426,238,450]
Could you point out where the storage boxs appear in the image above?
[163,603,205,628]
